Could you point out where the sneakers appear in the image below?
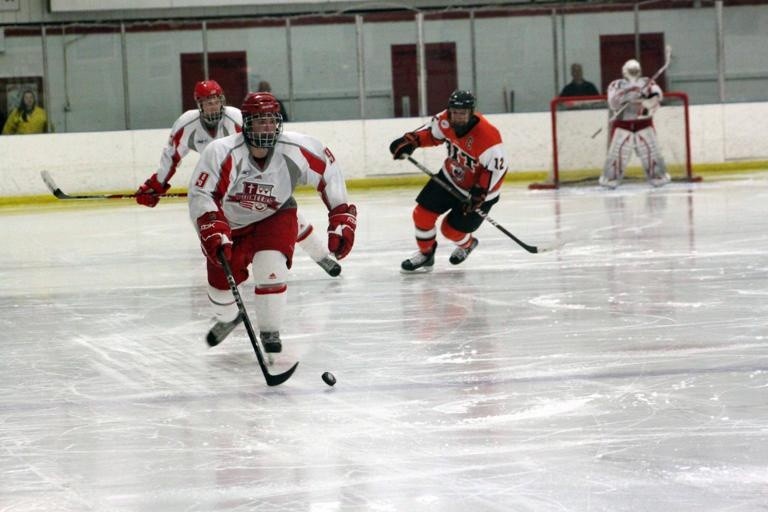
[260,331,282,351]
[401,240,437,271]
[318,256,341,277]
[450,238,479,264]
[206,311,243,347]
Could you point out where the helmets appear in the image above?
[195,80,226,127]
[241,93,283,149]
[622,59,642,84]
[448,90,476,128]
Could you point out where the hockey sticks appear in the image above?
[592,44,670,139]
[40,170,188,200]
[216,245,299,387]
[401,152,557,253]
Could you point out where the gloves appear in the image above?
[137,173,171,207]
[327,204,357,259]
[198,211,233,266]
[462,184,487,216]
[390,132,419,160]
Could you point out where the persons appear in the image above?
[186,92,359,357]
[602,61,676,189]
[389,89,510,275]
[561,64,600,98]
[134,81,346,280]
[255,78,290,121]
[2,89,54,135]
